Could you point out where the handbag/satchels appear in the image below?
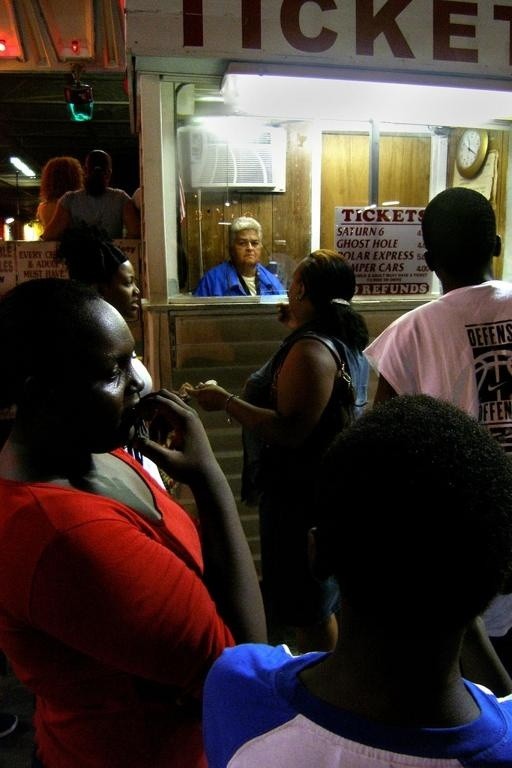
[240,368,353,490]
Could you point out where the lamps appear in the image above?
[65,62,95,122]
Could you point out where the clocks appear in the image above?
[454,127,489,180]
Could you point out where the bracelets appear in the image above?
[223,393,240,426]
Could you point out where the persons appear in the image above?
[198,388,512,767]
[36,154,85,231]
[60,240,171,502]
[361,186,510,675]
[192,214,290,297]
[181,245,368,654]
[38,143,142,243]
[0,274,276,766]
